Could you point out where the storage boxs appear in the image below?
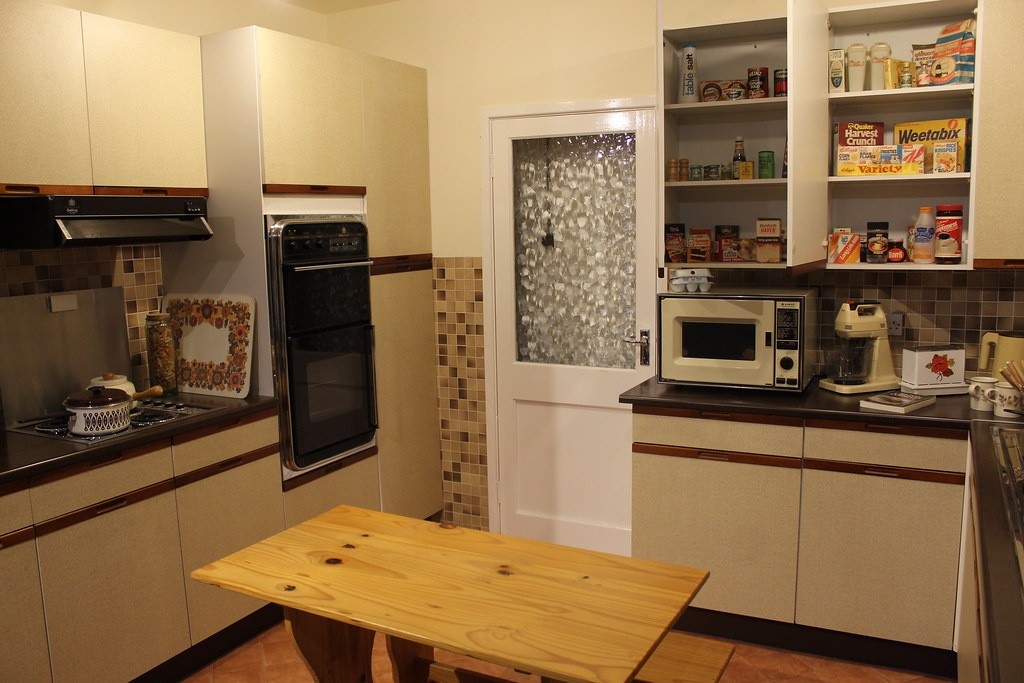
[933,141,957,173]
[831,142,926,175]
[831,120,884,146]
[894,116,973,173]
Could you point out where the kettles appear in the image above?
[977,329,1024,381]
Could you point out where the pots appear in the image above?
[85,371,164,411]
[64,387,135,437]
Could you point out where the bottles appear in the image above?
[670,134,747,181]
[915,205,936,264]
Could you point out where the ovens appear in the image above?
[257,205,386,475]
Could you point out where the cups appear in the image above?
[983,381,1022,418]
[969,374,998,411]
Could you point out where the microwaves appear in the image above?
[655,283,823,396]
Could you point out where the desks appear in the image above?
[184,499,719,681]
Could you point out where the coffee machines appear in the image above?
[819,300,902,395]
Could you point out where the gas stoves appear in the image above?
[8,400,211,447]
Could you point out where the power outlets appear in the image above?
[885,313,904,335]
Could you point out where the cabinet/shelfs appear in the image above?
[657,0,988,272]
[630,401,806,650]
[29,436,194,683]
[794,405,970,679]
[171,406,292,674]
[200,25,443,544]
[0,0,209,196]
[0,471,54,682]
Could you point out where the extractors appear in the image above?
[1,191,217,300]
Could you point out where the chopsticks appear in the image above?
[999,357,1024,396]
